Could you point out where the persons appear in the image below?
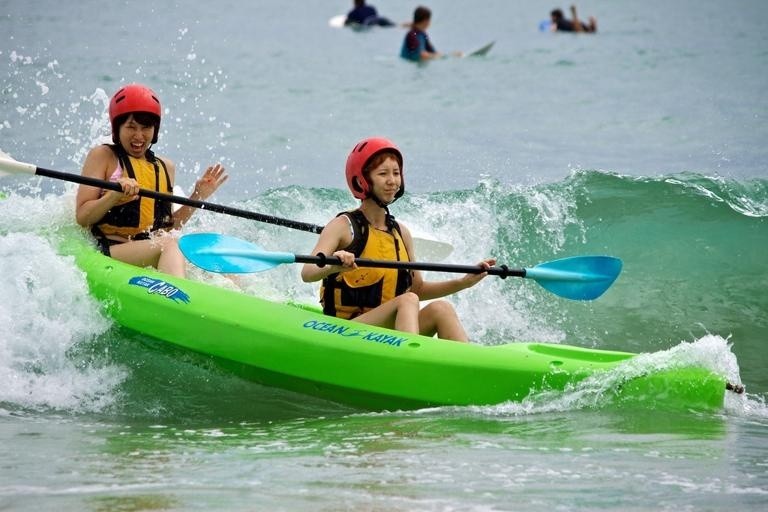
[551,6,597,31]
[345,0,394,26]
[301,137,497,345]
[400,6,464,63]
[76,84,229,279]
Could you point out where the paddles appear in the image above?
[2,151,453,265]
[178,233,622,299]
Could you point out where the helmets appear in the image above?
[109,84,161,144]
[345,137,404,200]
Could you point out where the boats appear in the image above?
[57,237,726,412]
